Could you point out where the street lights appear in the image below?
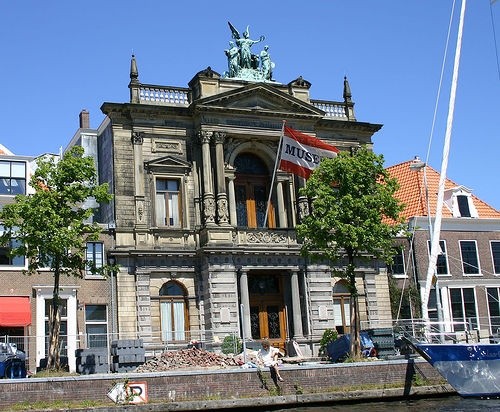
[410,156,445,343]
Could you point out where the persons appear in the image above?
[224,41,239,78]
[260,45,271,79]
[233,32,261,68]
[258,341,284,382]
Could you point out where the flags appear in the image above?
[278,126,340,187]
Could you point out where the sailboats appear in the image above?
[393,0,499,400]
